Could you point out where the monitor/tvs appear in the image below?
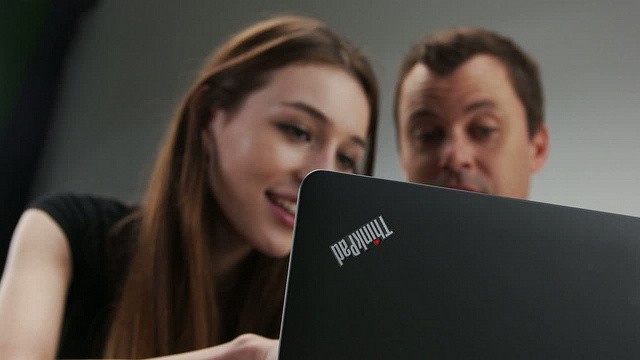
[276,168,640,360]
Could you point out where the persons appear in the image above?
[1,16,381,359]
[390,27,552,198]
